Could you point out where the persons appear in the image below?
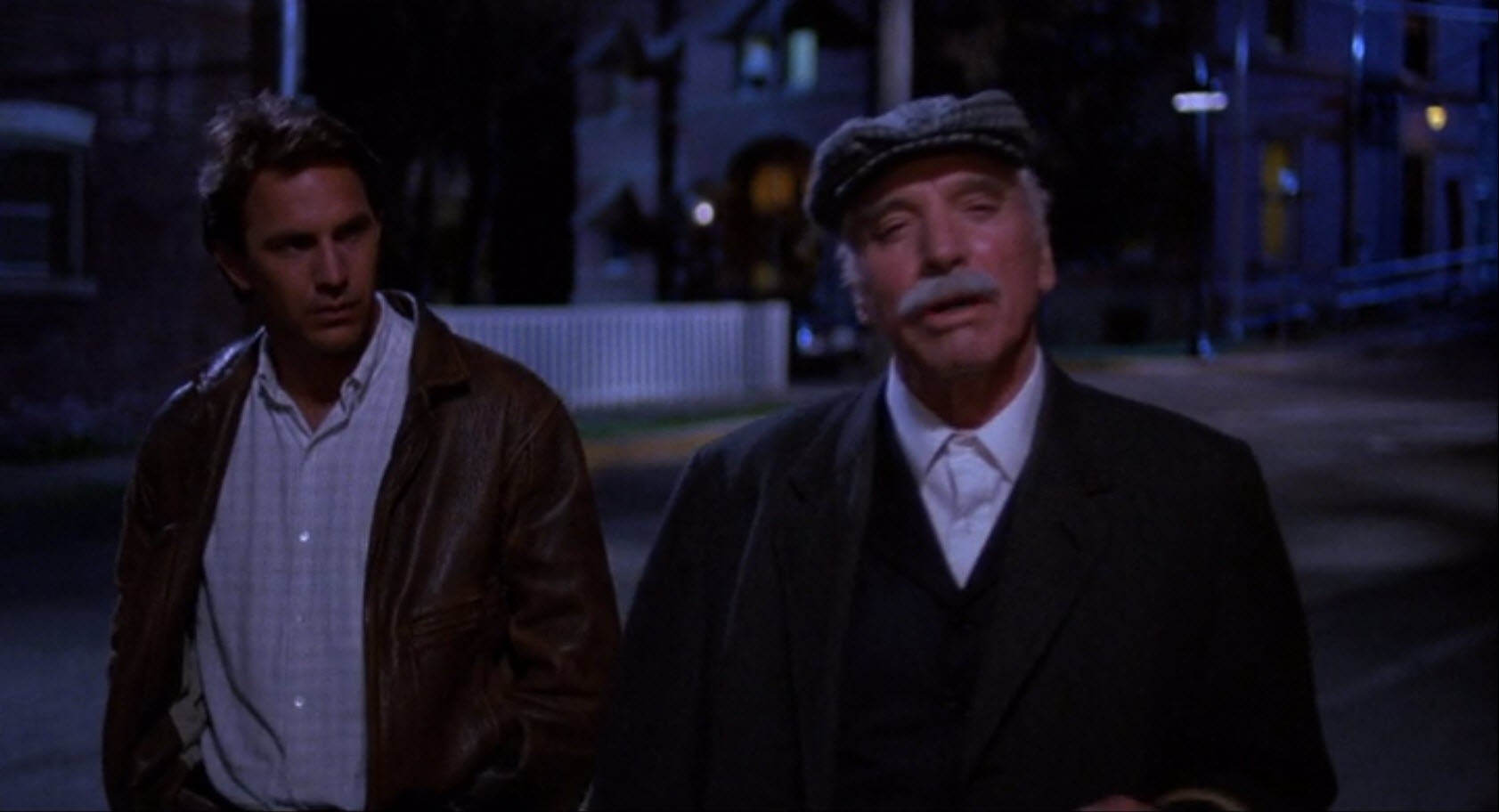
[587,91,1337,812]
[103,94,619,812]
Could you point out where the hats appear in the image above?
[801,89,1039,239]
